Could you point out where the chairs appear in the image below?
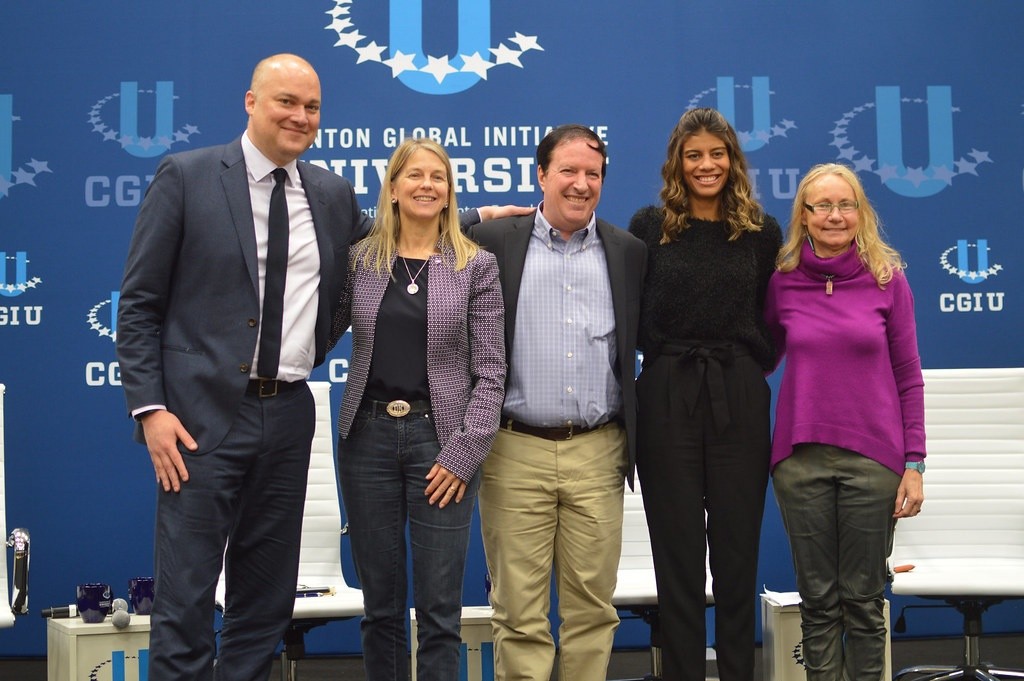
[885,368,1024,681]
[612,464,715,681]
[214,381,365,681]
[1,383,30,628]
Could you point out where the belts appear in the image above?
[499,414,613,441]
[362,399,431,417]
[246,379,306,397]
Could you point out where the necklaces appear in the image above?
[819,273,836,295]
[399,237,429,294]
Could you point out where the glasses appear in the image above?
[803,201,858,214]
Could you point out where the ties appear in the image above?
[256,168,290,379]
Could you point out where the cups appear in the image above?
[76,583,111,623]
[129,577,155,615]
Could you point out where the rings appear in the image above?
[449,486,457,491]
[917,509,920,512]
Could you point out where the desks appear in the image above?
[410,606,494,681]
[47,613,151,681]
[761,595,891,681]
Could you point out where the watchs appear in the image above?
[905,461,925,473]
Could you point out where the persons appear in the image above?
[117,53,927,681]
